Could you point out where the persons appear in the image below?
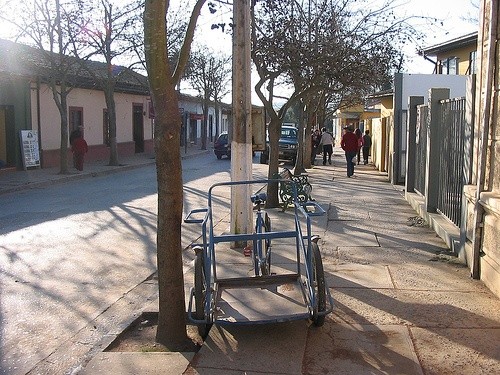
[70,124,88,170]
[341,125,357,176]
[310,126,373,166]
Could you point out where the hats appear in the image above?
[321,127,326,132]
[347,125,354,131]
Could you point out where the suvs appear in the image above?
[260,124,301,166]
[212,132,257,160]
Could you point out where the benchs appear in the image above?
[278,168,315,213]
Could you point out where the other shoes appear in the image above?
[347,174,351,178]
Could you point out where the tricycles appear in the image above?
[183,178,334,337]
